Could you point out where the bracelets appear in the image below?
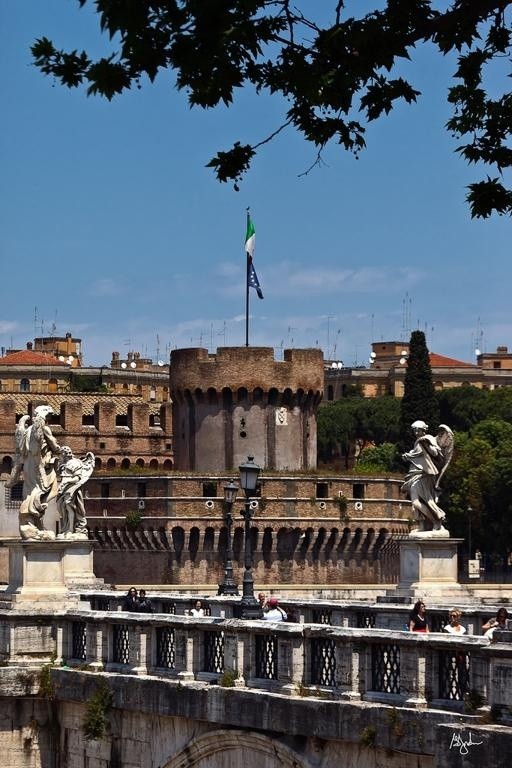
[490,624,493,627]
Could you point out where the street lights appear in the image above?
[221,478,238,596]
[236,455,260,612]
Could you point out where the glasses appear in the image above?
[450,615,456,617]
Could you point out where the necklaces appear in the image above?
[417,613,425,620]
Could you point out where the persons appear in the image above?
[255,591,270,619]
[506,551,512,576]
[400,419,450,537]
[34,442,87,534]
[13,404,61,539]
[409,600,431,632]
[440,609,471,694]
[121,586,140,611]
[263,597,289,620]
[480,607,512,632]
[133,588,153,612]
[474,547,486,570]
[189,599,205,616]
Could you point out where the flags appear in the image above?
[245,204,265,301]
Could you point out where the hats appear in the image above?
[270,598,277,606]
